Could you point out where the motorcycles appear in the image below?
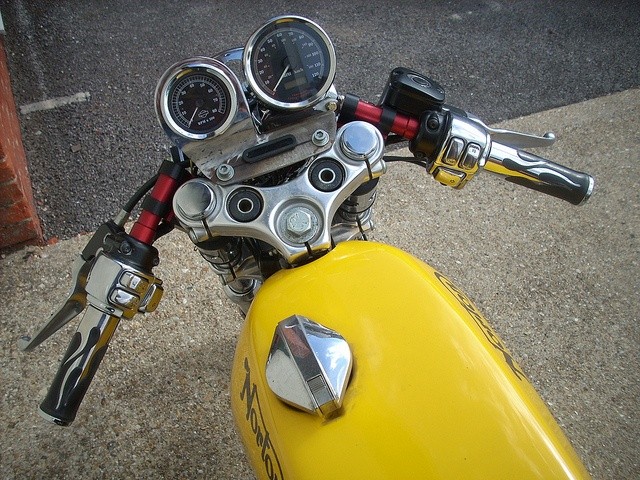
[21,14,594,480]
